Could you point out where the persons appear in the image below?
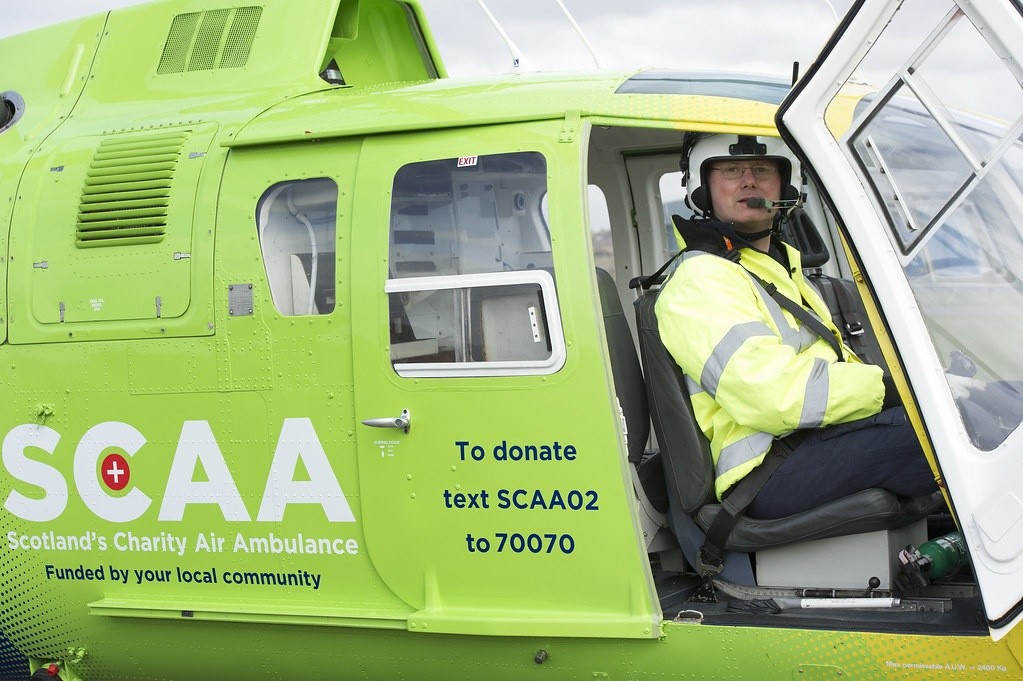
[654,135,1023,520]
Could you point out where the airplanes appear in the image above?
[0,0,1023,679]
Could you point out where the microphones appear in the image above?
[747,197,804,212]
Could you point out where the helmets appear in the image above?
[684,132,803,219]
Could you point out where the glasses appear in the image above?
[715,165,777,178]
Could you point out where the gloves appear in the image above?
[944,369,986,400]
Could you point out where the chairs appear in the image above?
[538,266,682,554]
[633,290,947,552]
[803,274,905,410]
